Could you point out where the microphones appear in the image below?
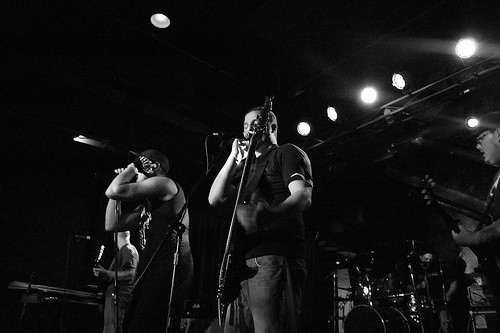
[76,236,90,239]
[212,132,252,140]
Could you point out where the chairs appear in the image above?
[342,304,387,333]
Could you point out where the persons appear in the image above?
[407,112,500,333]
[93,231,140,333]
[105,149,194,333]
[208,106,313,333]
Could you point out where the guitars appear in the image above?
[217,98,274,308]
[93,245,107,315]
[420,176,490,271]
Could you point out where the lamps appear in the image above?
[392,71,416,94]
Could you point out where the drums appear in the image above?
[343,304,410,333]
[403,294,435,313]
